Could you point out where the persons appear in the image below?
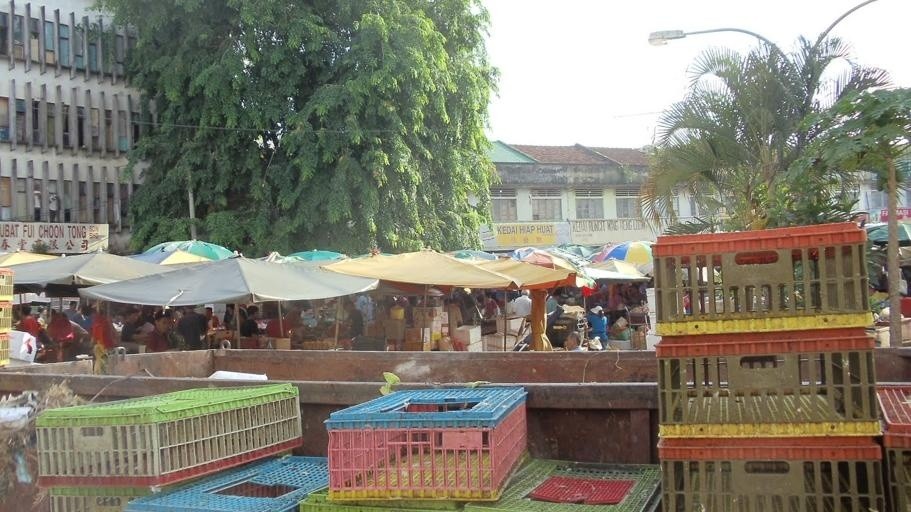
[2,235,721,367]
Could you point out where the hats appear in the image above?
[591,306,602,314]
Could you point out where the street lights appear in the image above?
[648,0,911,229]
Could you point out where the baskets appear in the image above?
[651,221,911,512]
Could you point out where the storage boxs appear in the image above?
[386,301,585,351]
[260,336,291,350]
[239,335,256,349]
[9,330,37,363]
[214,330,241,349]
